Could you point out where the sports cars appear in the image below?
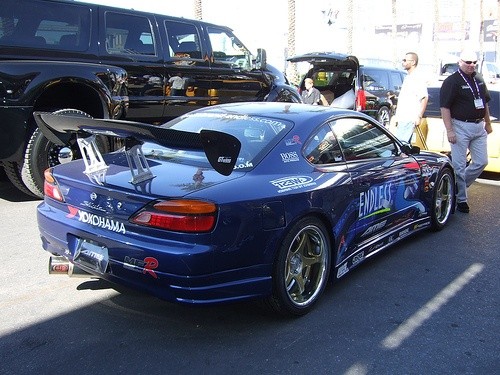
[33,102,458,319]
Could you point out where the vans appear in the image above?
[438,60,500,92]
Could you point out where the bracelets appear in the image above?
[418,116,422,119]
[448,129,453,131]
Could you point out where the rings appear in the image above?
[450,140,451,141]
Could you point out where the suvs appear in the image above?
[0,1,303,196]
[286,50,407,126]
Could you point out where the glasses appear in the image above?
[402,58,413,62]
[460,58,477,64]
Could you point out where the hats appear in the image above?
[304,78,313,84]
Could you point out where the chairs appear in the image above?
[31,35,47,49]
[241,127,266,148]
[59,33,77,46]
[176,41,198,53]
[135,40,153,53]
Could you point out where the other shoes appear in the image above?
[456,201,469,213]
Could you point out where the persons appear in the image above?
[143,71,163,97]
[301,78,320,105]
[439,50,493,214]
[167,71,210,105]
[388,51,428,148]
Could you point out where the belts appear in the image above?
[451,113,485,123]
